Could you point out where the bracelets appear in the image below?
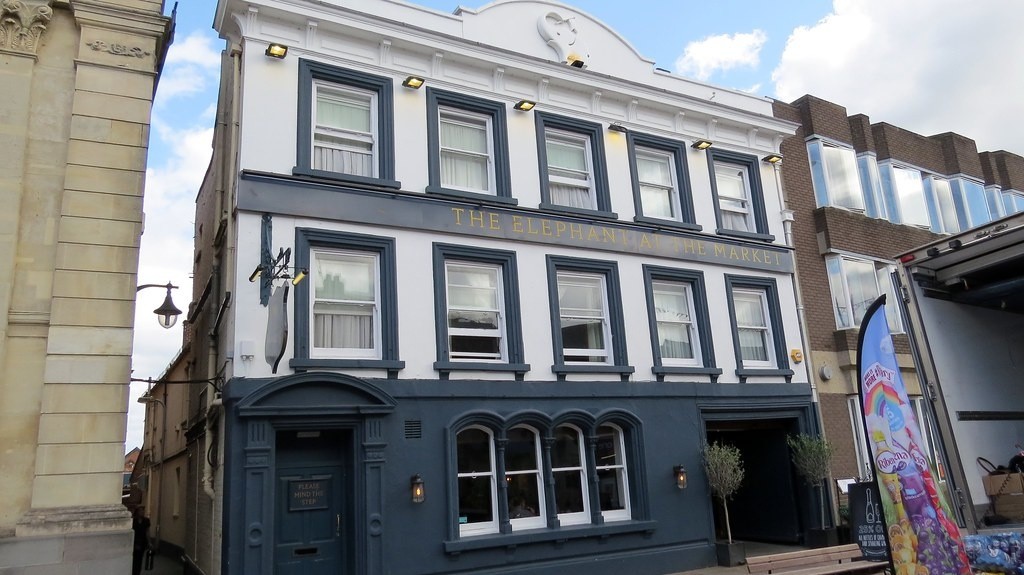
[529,507,531,510]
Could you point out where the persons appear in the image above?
[132,504,154,575]
[464,475,582,522]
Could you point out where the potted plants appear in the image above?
[836,504,855,546]
[700,439,746,567]
[787,432,838,549]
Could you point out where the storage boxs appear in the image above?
[982,472,1024,520]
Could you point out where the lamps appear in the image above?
[673,463,688,489]
[513,99,536,112]
[571,60,584,68]
[137,280,182,329]
[409,473,425,504]
[265,43,288,59]
[402,76,425,89]
[761,152,783,164]
[690,138,712,150]
[248,214,311,306]
[607,122,627,133]
